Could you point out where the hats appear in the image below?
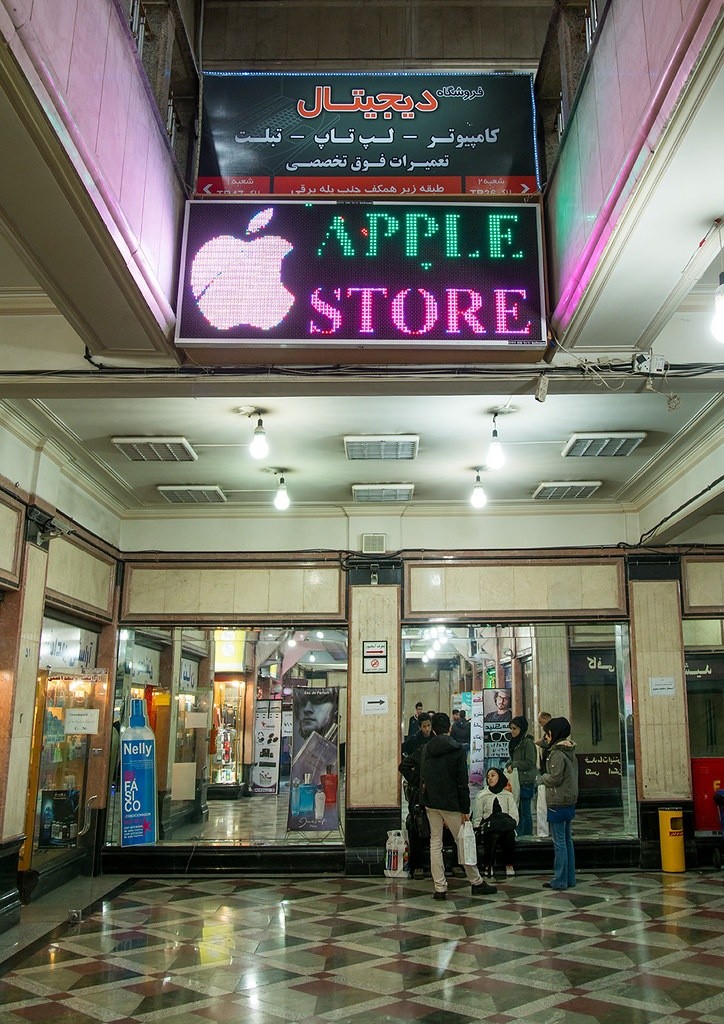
[494,689,511,698]
[453,710,460,715]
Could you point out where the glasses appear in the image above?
[296,694,333,707]
[497,695,509,702]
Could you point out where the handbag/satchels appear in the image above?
[456,820,478,865]
[383,829,411,878]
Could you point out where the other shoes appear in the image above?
[434,891,446,901]
[471,880,497,895]
[483,865,494,876]
[505,865,515,876]
[542,882,552,888]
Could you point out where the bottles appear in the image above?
[122,699,156,846]
[291,765,337,820]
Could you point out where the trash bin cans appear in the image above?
[658,807,686,872]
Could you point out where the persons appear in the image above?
[483,689,511,722]
[398,713,497,901]
[294,687,338,743]
[504,715,538,837]
[398,701,471,877]
[471,767,520,876]
[535,717,579,890]
[533,711,552,775]
